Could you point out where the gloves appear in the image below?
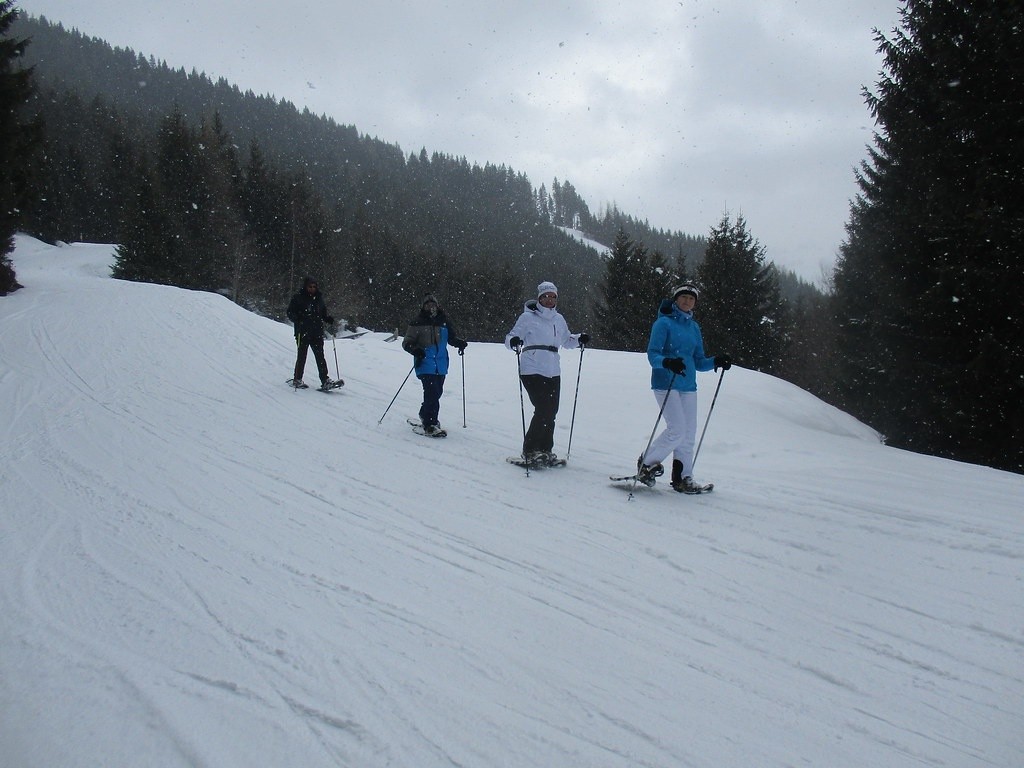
[455,339,468,349]
[510,337,523,351]
[412,346,425,358]
[715,352,732,370]
[323,315,333,323]
[663,358,686,374]
[578,333,589,343]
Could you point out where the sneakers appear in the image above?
[674,477,700,494]
[637,454,656,487]
[520,451,549,465]
[544,450,557,461]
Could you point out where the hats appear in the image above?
[423,292,437,307]
[537,282,557,299]
[673,282,698,301]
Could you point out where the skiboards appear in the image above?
[609,476,714,494]
[407,417,447,438]
[506,453,567,470]
[286,376,343,391]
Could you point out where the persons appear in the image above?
[402,293,468,433]
[287,278,335,388]
[505,281,590,463]
[637,283,732,494]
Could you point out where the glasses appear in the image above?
[308,285,316,288]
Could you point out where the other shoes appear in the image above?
[418,413,440,427]
[293,379,304,387]
[322,378,335,388]
[425,425,442,435]
